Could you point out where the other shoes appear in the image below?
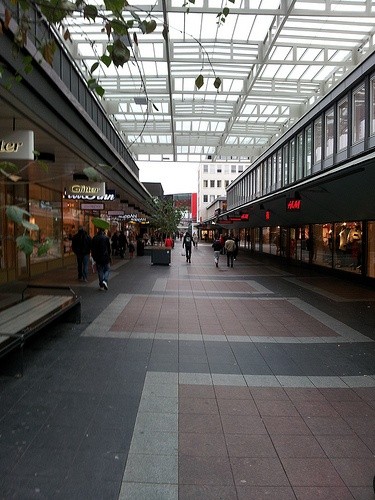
[102,281,109,291]
[99,287,104,291]
[78,278,82,282]
[82,278,88,283]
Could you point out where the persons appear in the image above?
[108,230,183,258]
[127,241,136,260]
[182,232,195,263]
[90,228,111,290]
[224,236,236,268]
[212,237,223,267]
[72,225,93,283]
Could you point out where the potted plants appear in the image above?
[145,197,188,264]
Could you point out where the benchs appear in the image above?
[0,285,80,358]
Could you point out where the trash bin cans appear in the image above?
[151,248,171,266]
